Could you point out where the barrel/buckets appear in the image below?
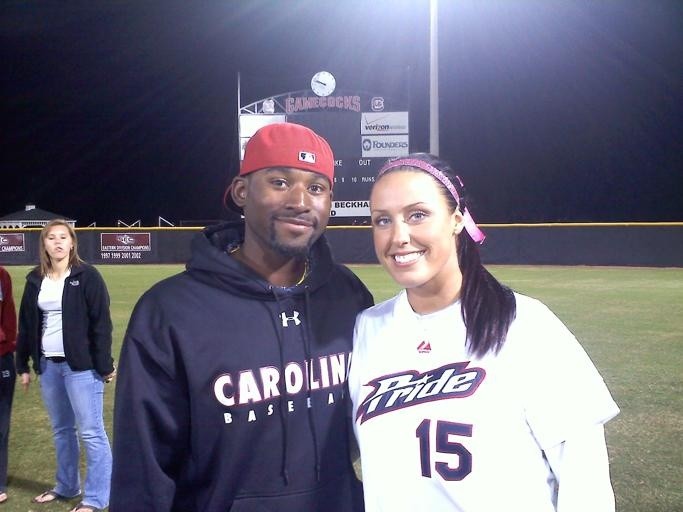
[69,503,99,512]
[33,490,61,503]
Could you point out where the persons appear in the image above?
[13,218,117,511]
[105,122,373,512]
[0,265,18,504]
[345,150,622,512]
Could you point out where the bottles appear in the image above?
[222,122,335,212]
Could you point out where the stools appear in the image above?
[44,356,67,363]
